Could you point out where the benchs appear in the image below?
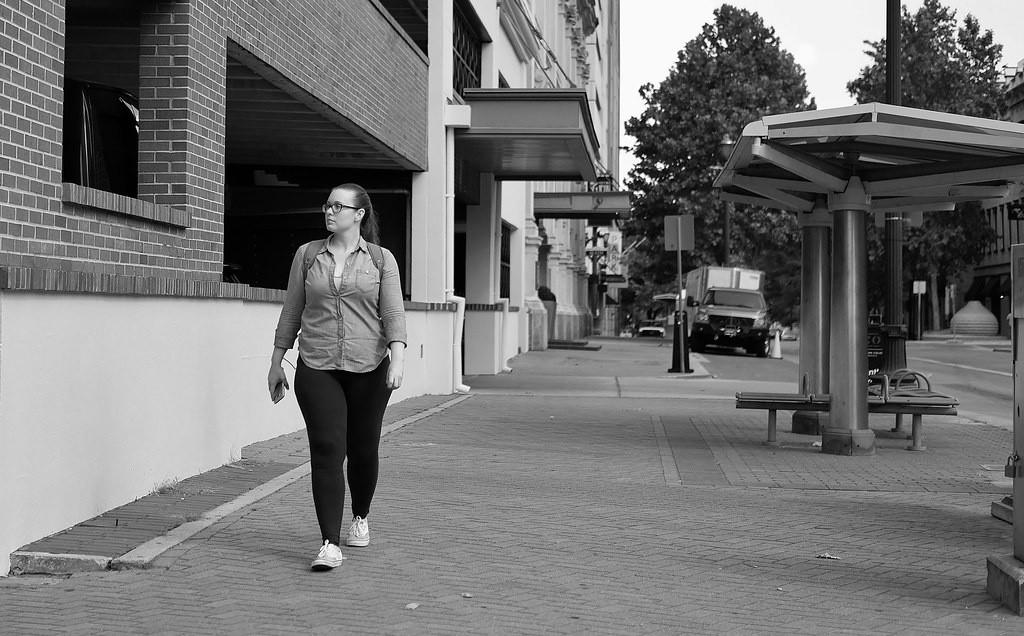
[735,368,960,451]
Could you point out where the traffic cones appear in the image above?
[770,330,784,359]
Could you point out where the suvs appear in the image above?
[686,285,773,358]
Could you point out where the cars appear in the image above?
[639,320,666,337]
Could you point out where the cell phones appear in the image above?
[272,367,286,404]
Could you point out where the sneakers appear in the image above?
[345,515,370,546]
[311,539,343,570]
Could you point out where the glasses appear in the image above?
[322,204,360,213]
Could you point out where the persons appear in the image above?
[268,183,410,572]
